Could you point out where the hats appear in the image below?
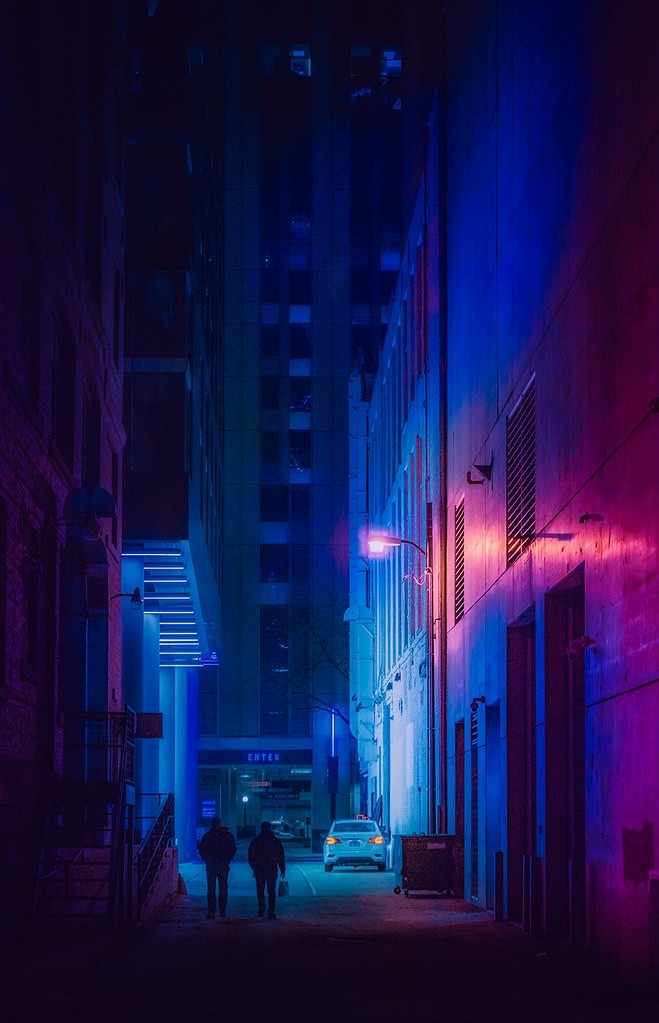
[211,817,221,822]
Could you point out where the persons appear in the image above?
[247,822,286,917]
[199,817,237,918]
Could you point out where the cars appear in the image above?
[320,818,386,872]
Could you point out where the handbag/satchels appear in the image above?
[278,876,289,897]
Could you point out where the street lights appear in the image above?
[242,795,248,831]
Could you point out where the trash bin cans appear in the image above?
[392,832,461,898]
